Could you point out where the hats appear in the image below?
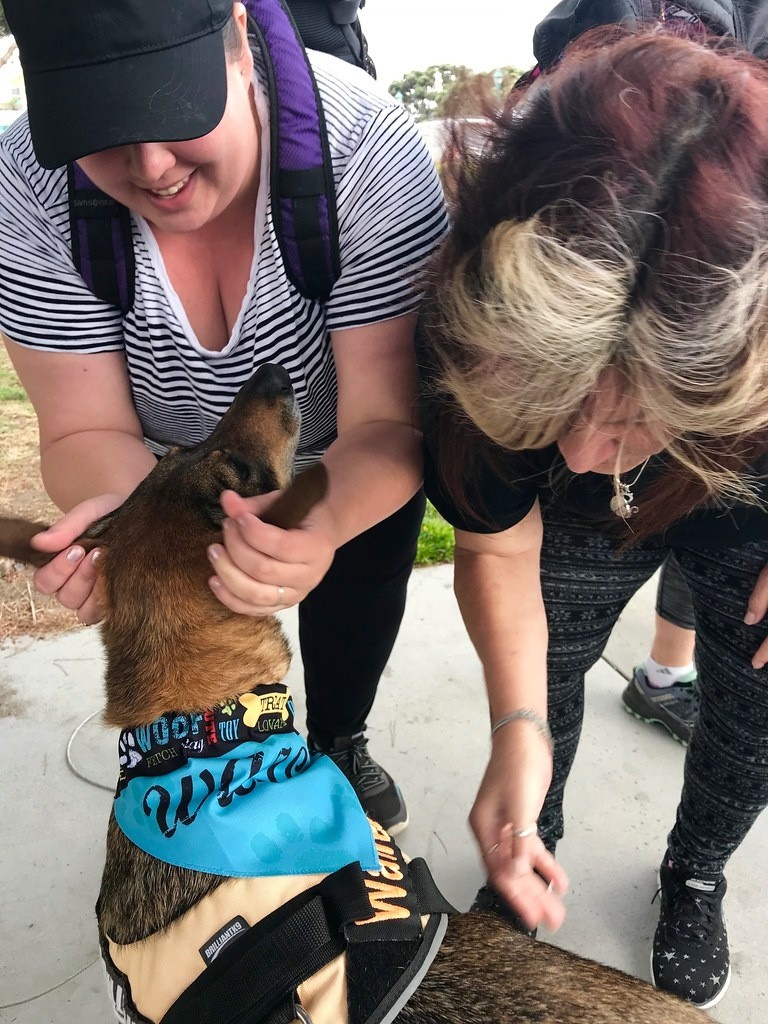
[0,0,236,169]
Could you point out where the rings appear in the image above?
[271,585,285,607]
[481,841,506,857]
[512,823,538,841]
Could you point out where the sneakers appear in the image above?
[651,846,731,1010]
[468,843,558,940]
[620,663,704,746]
[306,724,409,835]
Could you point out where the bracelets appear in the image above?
[490,707,555,754]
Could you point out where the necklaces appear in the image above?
[609,454,651,518]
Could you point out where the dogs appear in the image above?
[0,362,716,1024]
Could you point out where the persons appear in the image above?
[620,549,697,747]
[411,11,768,1012]
[0,0,454,840]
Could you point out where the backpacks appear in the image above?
[69,0,374,308]
